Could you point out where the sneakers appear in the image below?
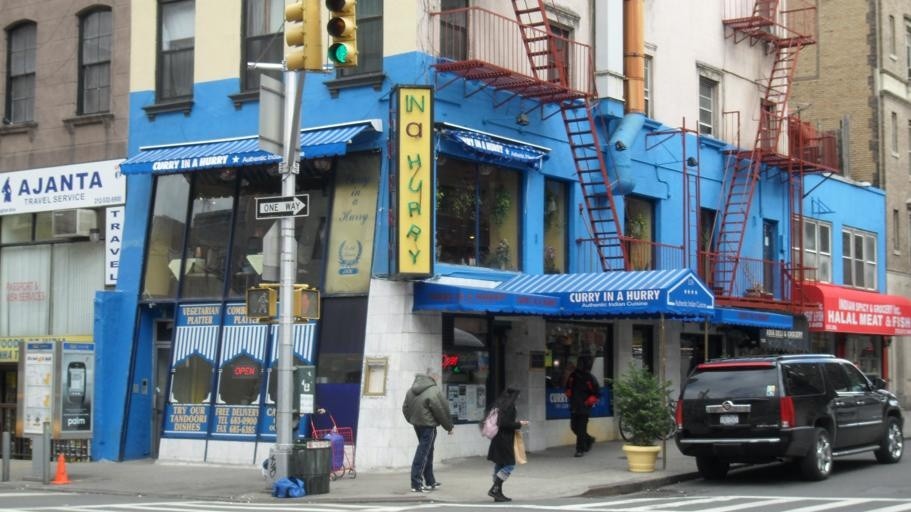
[411,482,441,492]
[574,436,596,457]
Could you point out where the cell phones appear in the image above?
[65,361,87,406]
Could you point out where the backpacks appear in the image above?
[481,407,498,439]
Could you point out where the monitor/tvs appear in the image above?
[51,209,97,238]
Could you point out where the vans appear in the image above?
[675,354,905,481]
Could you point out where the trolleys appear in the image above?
[301,408,356,481]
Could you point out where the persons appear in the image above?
[488,384,529,502]
[401,367,455,494]
[564,356,602,457]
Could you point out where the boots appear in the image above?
[488,481,512,502]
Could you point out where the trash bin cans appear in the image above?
[291,437,333,495]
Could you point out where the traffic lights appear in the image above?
[323,0,358,74]
[284,2,325,70]
[247,283,323,326]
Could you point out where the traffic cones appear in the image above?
[52,451,69,487]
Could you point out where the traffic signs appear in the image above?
[255,196,309,220]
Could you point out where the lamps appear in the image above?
[601,141,626,152]
[485,113,529,126]
[659,156,699,168]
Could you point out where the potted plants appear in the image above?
[603,358,676,473]
